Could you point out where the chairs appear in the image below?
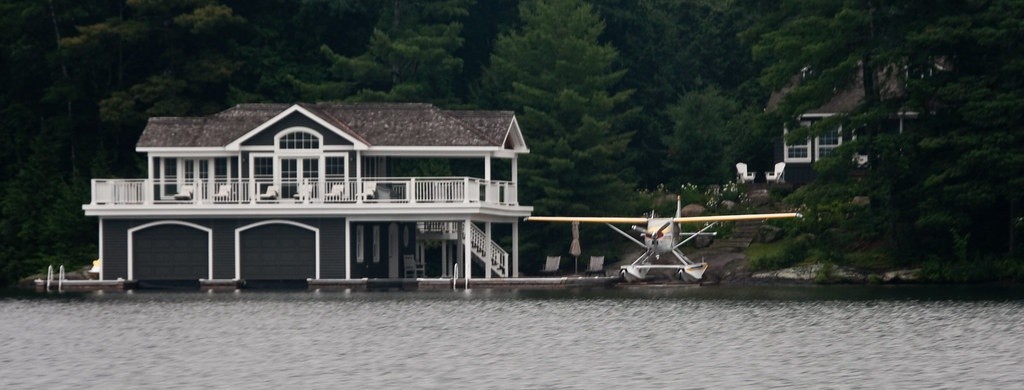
[356,181,377,199]
[325,184,344,199]
[215,185,232,201]
[585,256,606,277]
[404,255,424,278]
[174,185,193,200]
[764,162,786,184]
[87,259,100,278]
[294,184,312,204]
[736,162,757,184]
[539,256,562,277]
[261,186,279,200]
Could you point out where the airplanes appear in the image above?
[522,193,803,284]
